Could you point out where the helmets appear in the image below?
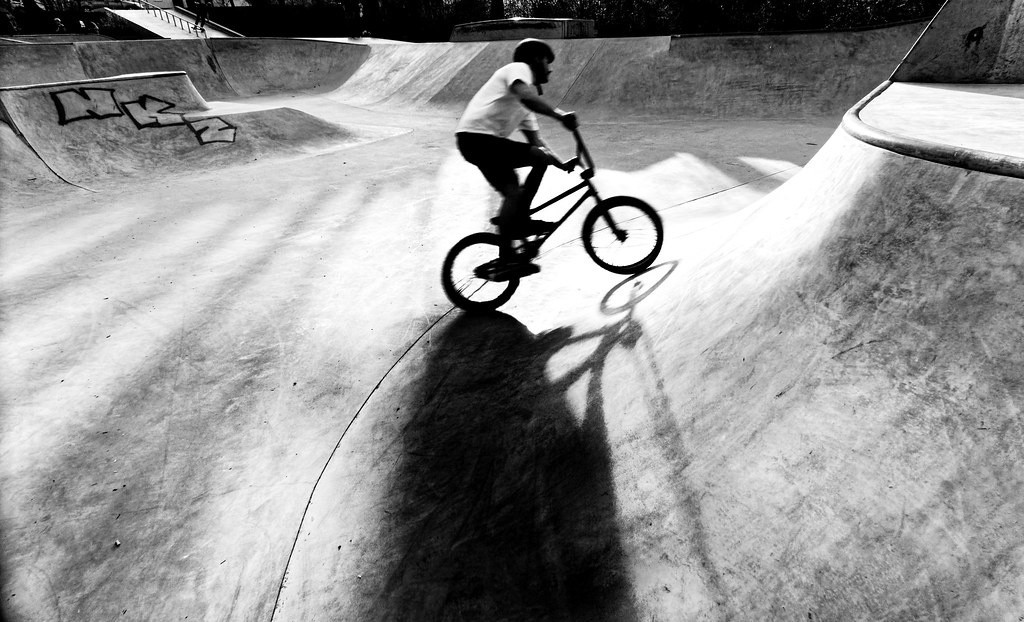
[512,37,555,64]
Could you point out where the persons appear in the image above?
[191,0,209,31]
[455,38,577,276]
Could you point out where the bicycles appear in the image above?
[439,131,665,314]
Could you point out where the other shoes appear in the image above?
[500,219,548,239]
